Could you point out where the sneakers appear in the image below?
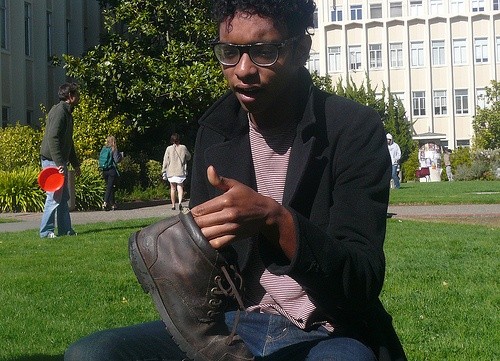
[128,207,258,361]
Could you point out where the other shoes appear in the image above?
[46,232,57,239]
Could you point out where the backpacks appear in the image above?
[99,147,113,169]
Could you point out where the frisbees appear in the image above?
[38,167,64,192]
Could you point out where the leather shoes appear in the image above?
[103,205,110,211]
[112,205,119,211]
[178,204,182,209]
[172,206,176,210]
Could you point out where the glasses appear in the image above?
[210,31,301,68]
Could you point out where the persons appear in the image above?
[386,133,402,188]
[63,1,408,361]
[39,83,81,238]
[161,133,191,210]
[444,148,453,181]
[99,136,123,211]
